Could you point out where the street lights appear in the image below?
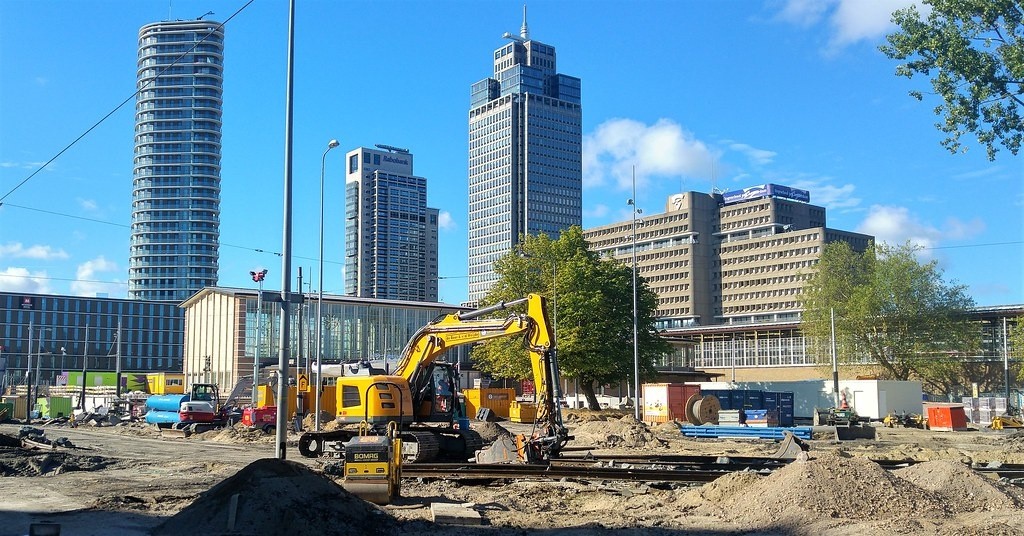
[315,139,340,433]
[626,163,643,422]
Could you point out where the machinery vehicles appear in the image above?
[170,372,281,434]
[298,291,571,465]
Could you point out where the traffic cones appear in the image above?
[841,392,849,408]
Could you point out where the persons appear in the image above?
[435,380,449,412]
